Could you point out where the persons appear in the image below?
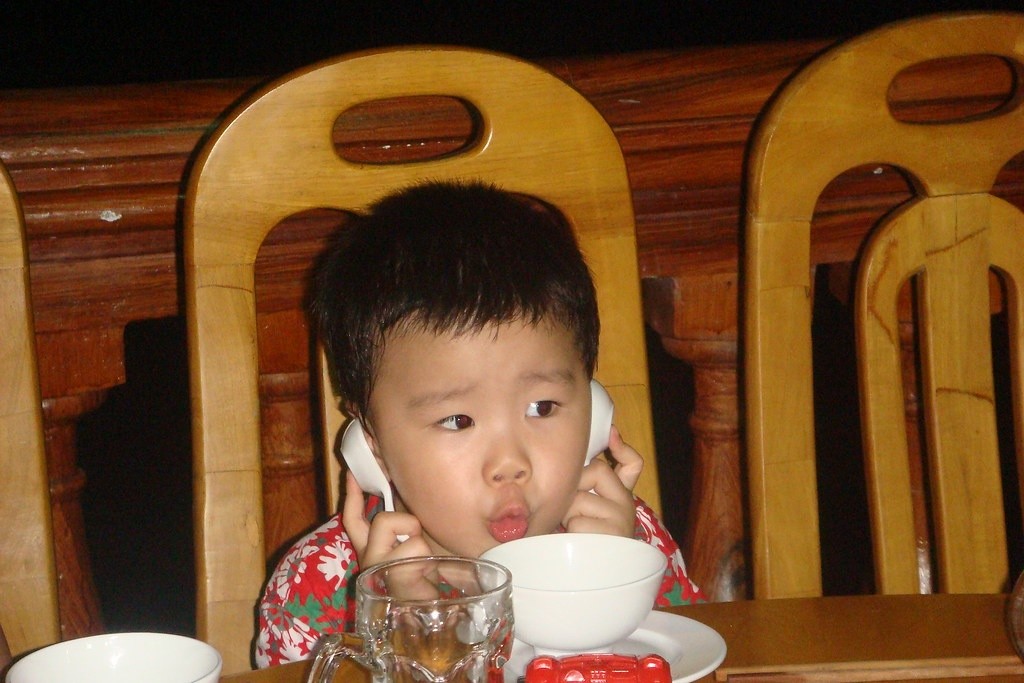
[253,182,709,670]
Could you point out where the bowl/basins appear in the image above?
[478,533,669,662]
[6,632,222,683]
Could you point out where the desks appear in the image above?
[218,590,1024,683]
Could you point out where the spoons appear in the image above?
[585,378,613,496]
[339,419,411,544]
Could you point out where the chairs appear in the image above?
[183,47,663,680]
[0,163,62,683]
[744,12,1024,599]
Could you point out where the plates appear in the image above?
[467,611,727,683]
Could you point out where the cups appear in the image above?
[306,555,512,683]
